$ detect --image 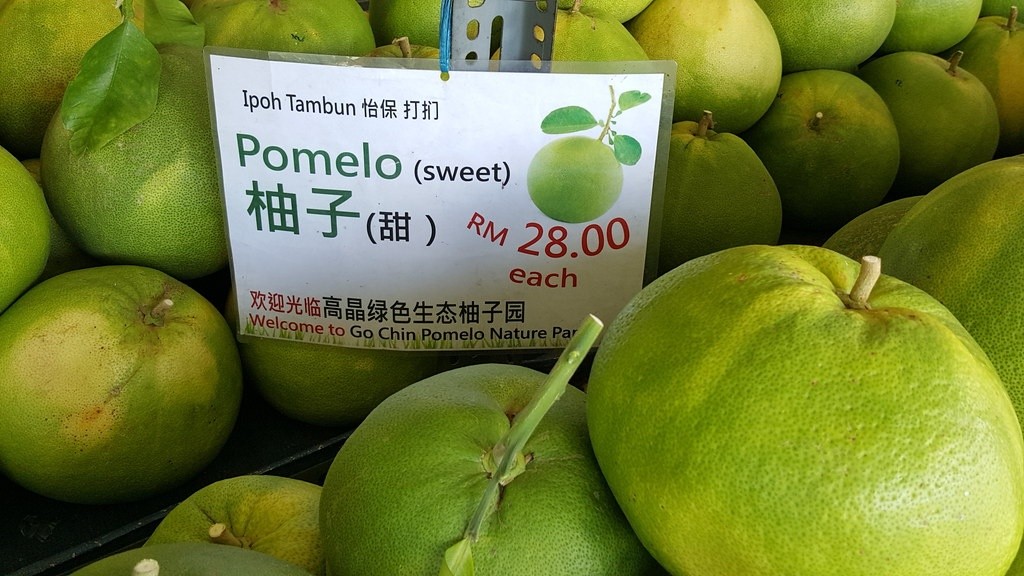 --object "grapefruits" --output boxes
[0,0,1024,576]
